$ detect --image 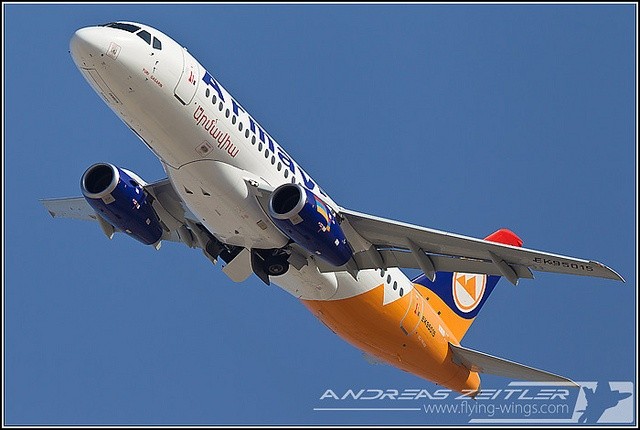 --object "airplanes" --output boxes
[38,20,626,401]
